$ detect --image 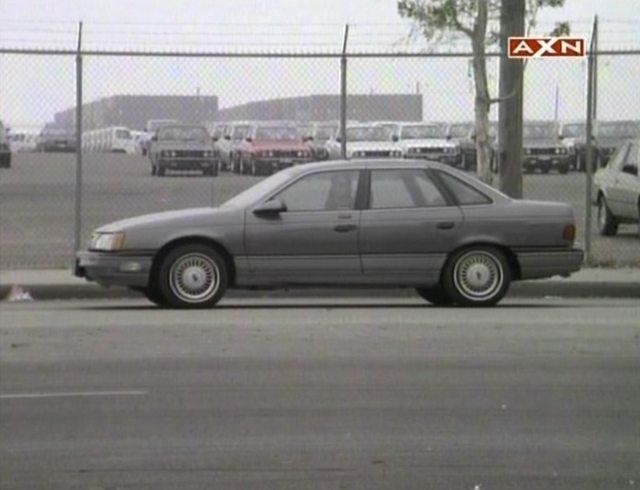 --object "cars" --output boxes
[1,118,15,171]
[70,154,590,313]
[591,135,640,236]
[79,118,640,176]
[38,129,74,153]
